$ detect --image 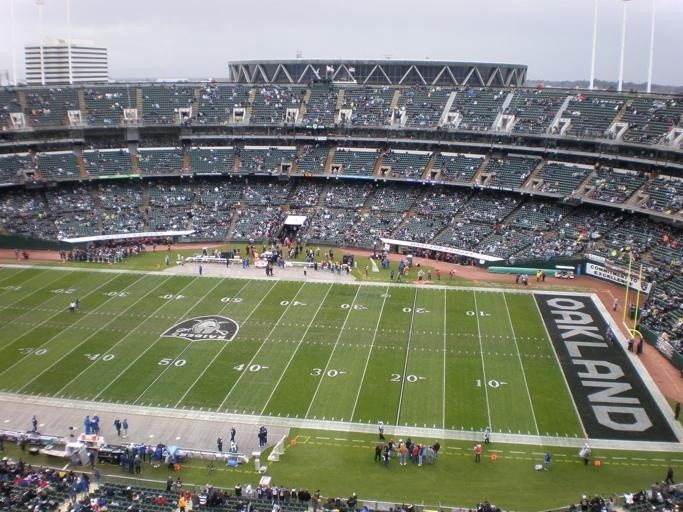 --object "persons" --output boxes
[1,84,682,286]
[612,299,618,311]
[675,402,680,419]
[70,298,80,312]
[627,335,643,355]
[2,413,682,512]
[639,286,682,354]
